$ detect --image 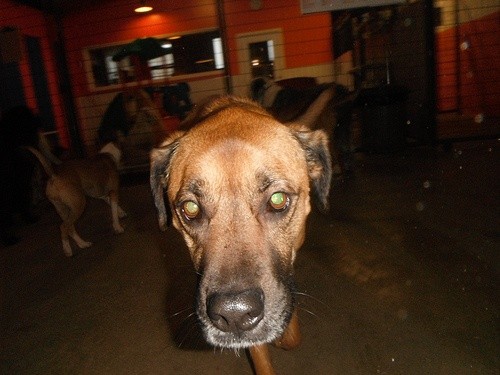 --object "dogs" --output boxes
[267,76,350,170]
[26,127,127,257]
[150,93,333,375]
